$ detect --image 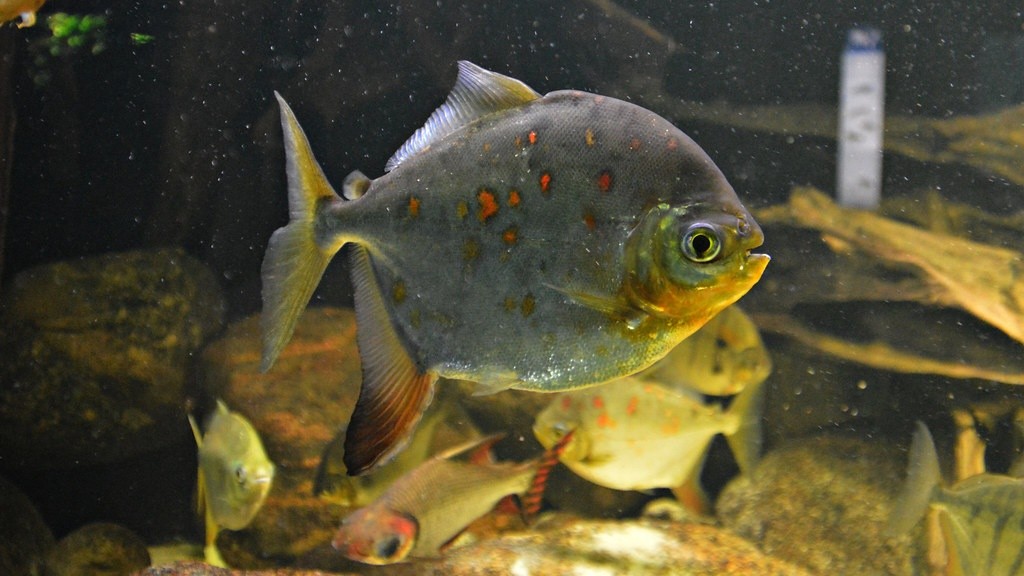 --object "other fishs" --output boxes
[187,62,1024,565]
